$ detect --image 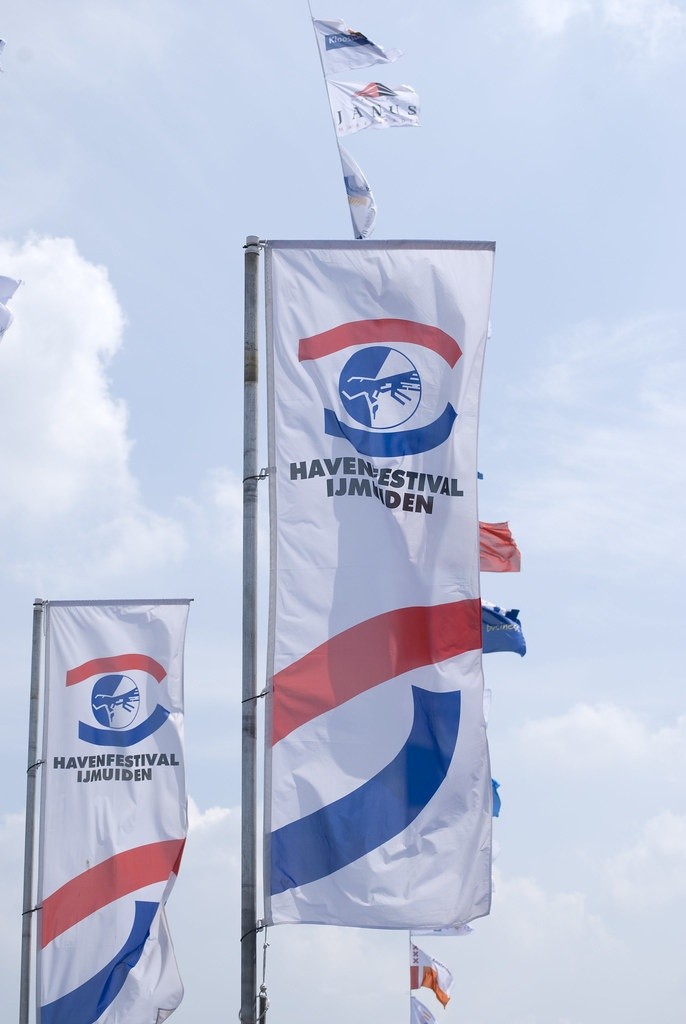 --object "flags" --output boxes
[313,14,422,131]
[41,597,191,1024]
[267,239,531,1023]
